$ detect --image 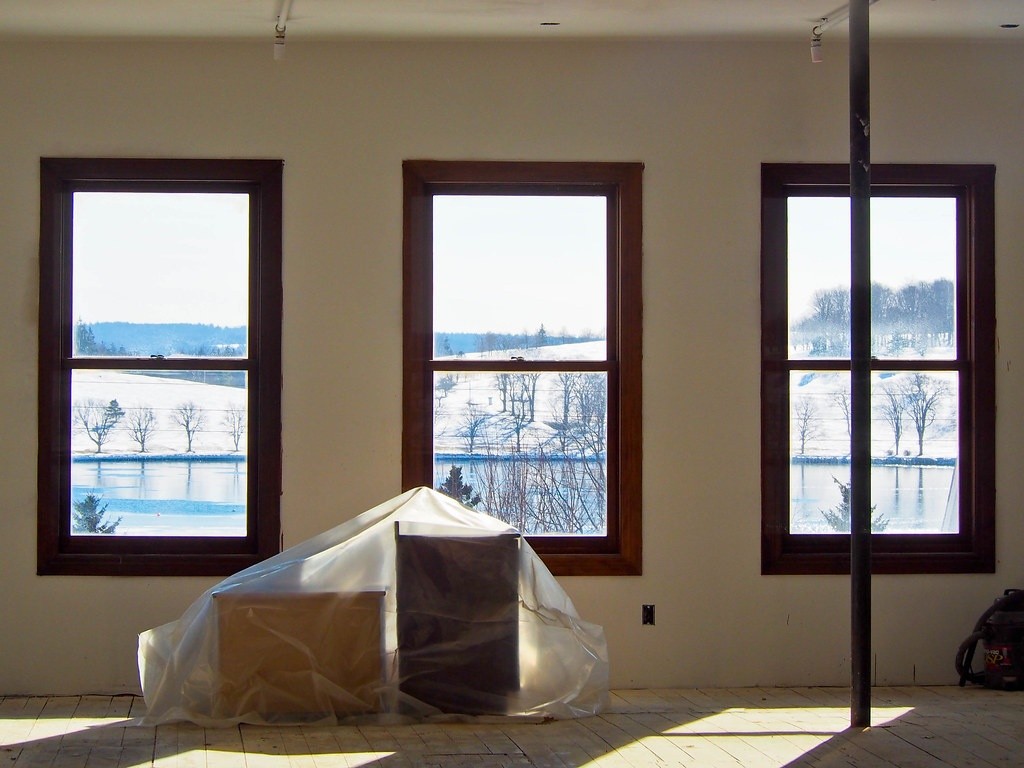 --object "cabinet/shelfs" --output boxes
[394,521,521,714]
[212,586,393,718]
